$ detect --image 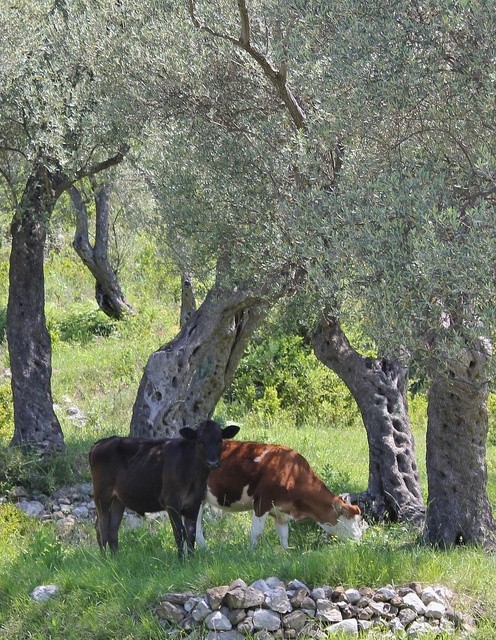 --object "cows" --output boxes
[89,421,240,566]
[195,440,370,551]
